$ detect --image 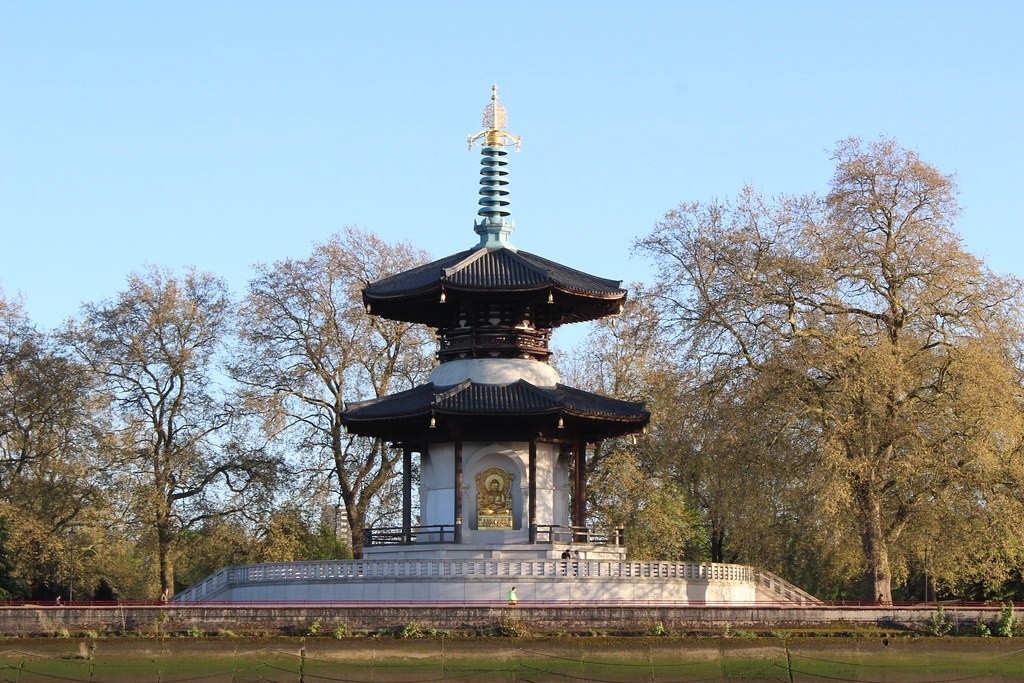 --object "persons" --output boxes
[159,588,168,603]
[562,549,581,576]
[54,595,63,606]
[878,594,884,606]
[508,587,518,605]
[481,479,509,516]
